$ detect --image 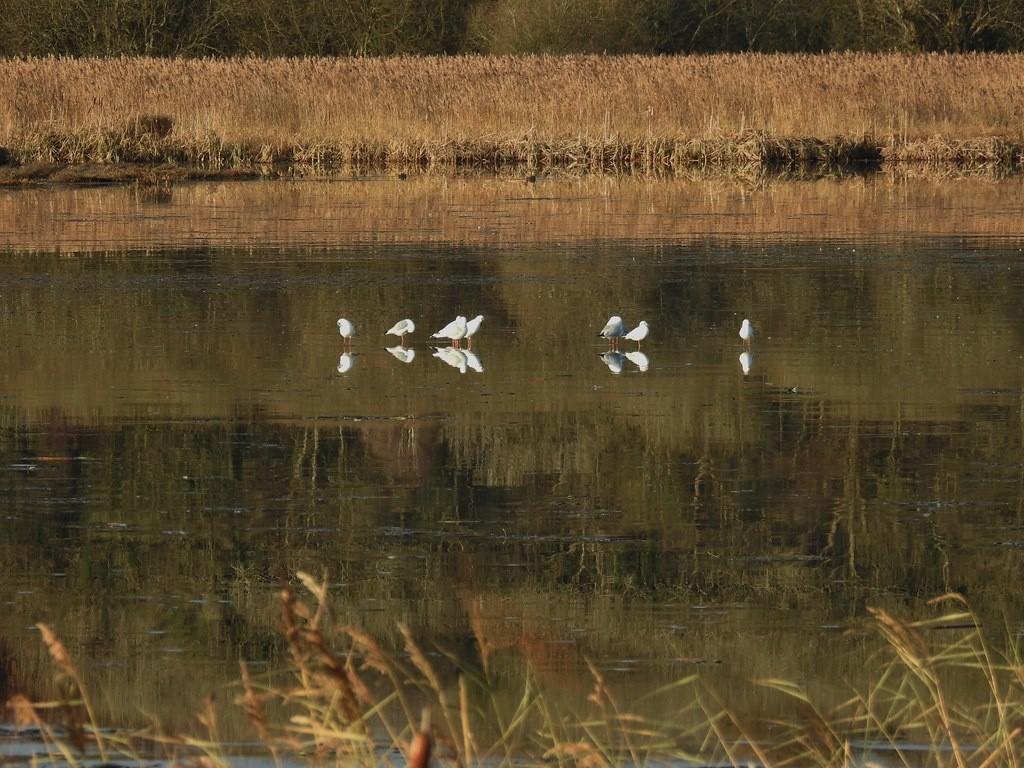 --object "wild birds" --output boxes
[336,319,357,344]
[597,348,649,374]
[380,317,416,340]
[337,344,355,373]
[429,312,484,344]
[739,346,756,374]
[380,340,416,363]
[430,344,484,374]
[739,318,755,346]
[597,314,649,347]
